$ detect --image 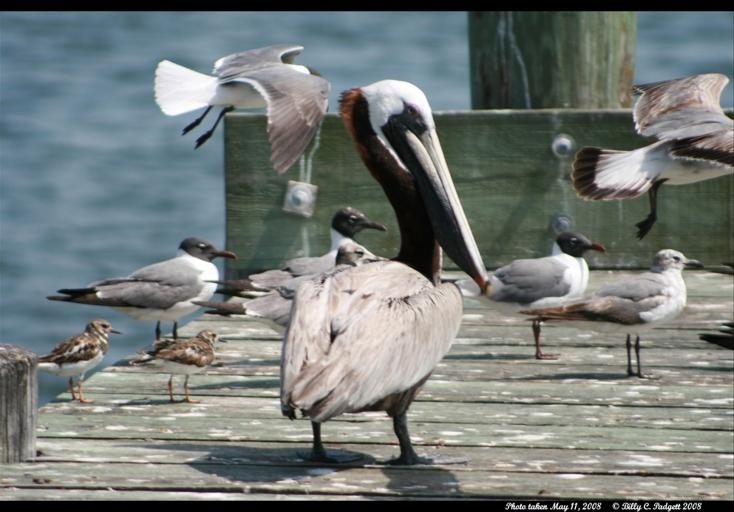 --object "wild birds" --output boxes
[45,237,237,340]
[569,73,733,241]
[191,206,389,339]
[454,232,608,360]
[128,328,227,403]
[35,318,123,403]
[519,249,704,380]
[280,79,491,465]
[153,44,330,175]
